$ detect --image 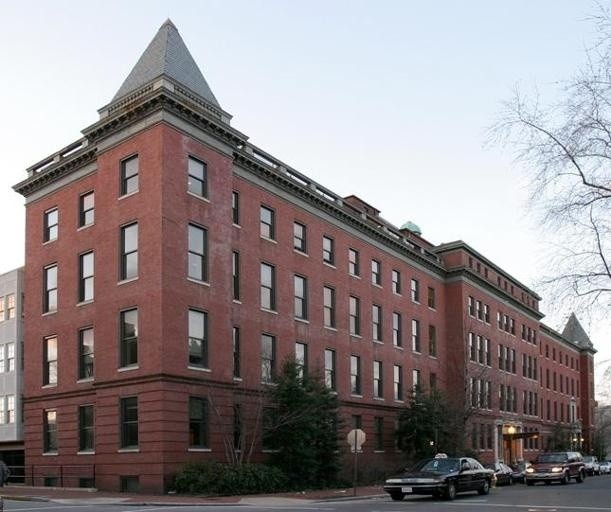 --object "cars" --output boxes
[383,452,611,500]
